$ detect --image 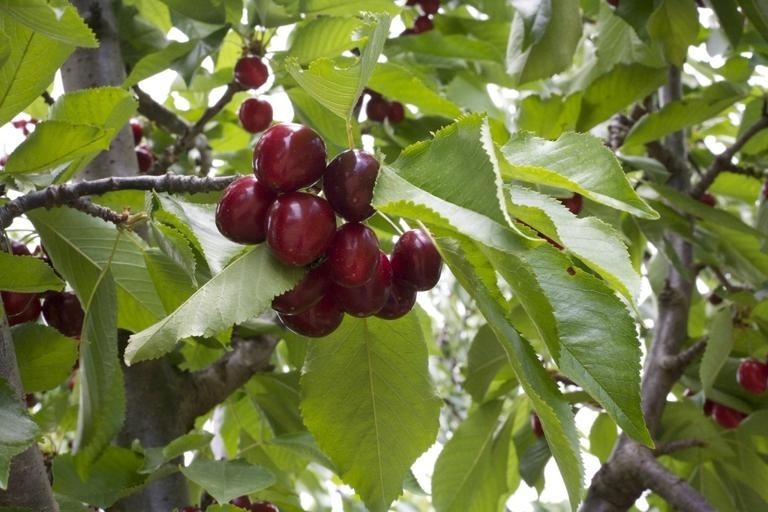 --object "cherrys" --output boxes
[0,0,768,512]
